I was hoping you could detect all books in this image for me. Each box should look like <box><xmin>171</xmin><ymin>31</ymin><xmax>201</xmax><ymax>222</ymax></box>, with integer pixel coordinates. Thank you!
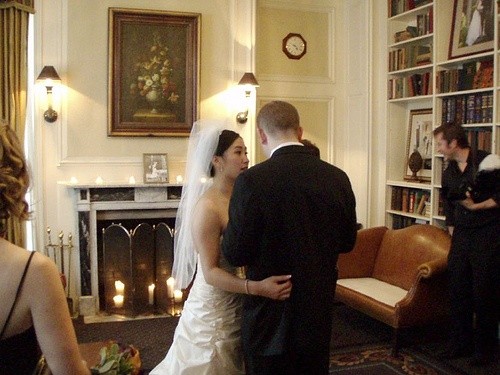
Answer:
<box><xmin>387</xmin><ymin>0</ymin><xmax>494</xmax><ymax>236</ymax></box>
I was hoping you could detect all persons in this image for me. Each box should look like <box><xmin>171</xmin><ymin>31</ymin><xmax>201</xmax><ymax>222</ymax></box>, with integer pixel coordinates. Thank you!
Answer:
<box><xmin>0</xmin><ymin>121</ymin><xmax>91</xmax><ymax>375</ymax></box>
<box><xmin>149</xmin><ymin>101</ymin><xmax>358</xmax><ymax>375</ymax></box>
<box><xmin>433</xmin><ymin>123</ymin><xmax>500</xmax><ymax>363</ymax></box>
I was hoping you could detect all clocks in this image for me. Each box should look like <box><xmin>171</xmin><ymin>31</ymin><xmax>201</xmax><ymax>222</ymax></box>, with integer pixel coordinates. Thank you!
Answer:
<box><xmin>281</xmin><ymin>32</ymin><xmax>307</xmax><ymax>60</ymax></box>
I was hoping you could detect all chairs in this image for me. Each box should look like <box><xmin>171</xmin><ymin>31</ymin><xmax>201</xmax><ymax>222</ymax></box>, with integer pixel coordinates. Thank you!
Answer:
<box><xmin>333</xmin><ymin>223</ymin><xmax>452</xmax><ymax>358</ymax></box>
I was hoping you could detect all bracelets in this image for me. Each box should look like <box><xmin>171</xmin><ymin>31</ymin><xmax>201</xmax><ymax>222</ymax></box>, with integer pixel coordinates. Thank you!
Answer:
<box><xmin>244</xmin><ymin>278</ymin><xmax>251</xmax><ymax>295</ymax></box>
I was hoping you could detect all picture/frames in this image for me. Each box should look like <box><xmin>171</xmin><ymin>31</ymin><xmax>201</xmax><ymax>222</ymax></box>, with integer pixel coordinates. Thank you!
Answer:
<box><xmin>403</xmin><ymin>108</ymin><xmax>433</xmax><ymax>183</ymax></box>
<box><xmin>448</xmin><ymin>0</ymin><xmax>495</xmax><ymax>61</ymax></box>
<box><xmin>104</xmin><ymin>6</ymin><xmax>203</xmax><ymax>139</ymax></box>
<box><xmin>143</xmin><ymin>152</ymin><xmax>169</xmax><ymax>184</ymax></box>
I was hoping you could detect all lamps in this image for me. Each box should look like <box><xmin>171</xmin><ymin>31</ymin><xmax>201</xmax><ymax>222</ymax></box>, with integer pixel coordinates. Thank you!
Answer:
<box><xmin>234</xmin><ymin>72</ymin><xmax>260</xmax><ymax>125</ymax></box>
<box><xmin>36</xmin><ymin>65</ymin><xmax>62</xmax><ymax>123</ymax></box>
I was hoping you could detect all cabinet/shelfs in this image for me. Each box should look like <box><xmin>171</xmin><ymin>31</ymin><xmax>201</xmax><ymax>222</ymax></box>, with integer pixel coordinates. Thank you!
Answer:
<box><xmin>385</xmin><ymin>0</ymin><xmax>500</xmax><ymax>230</ymax></box>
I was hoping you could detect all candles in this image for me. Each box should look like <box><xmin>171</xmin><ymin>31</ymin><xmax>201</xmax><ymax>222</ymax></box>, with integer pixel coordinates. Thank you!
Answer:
<box><xmin>165</xmin><ymin>278</ymin><xmax>175</xmax><ymax>299</ymax></box>
<box><xmin>113</xmin><ymin>295</ymin><xmax>124</xmax><ymax>308</ymax></box>
<box><xmin>172</xmin><ymin>290</ymin><xmax>183</xmax><ymax>303</ymax></box>
<box><xmin>114</xmin><ymin>280</ymin><xmax>125</xmax><ymax>295</ymax></box>
<box><xmin>147</xmin><ymin>284</ymin><xmax>155</xmax><ymax>305</ymax></box>
